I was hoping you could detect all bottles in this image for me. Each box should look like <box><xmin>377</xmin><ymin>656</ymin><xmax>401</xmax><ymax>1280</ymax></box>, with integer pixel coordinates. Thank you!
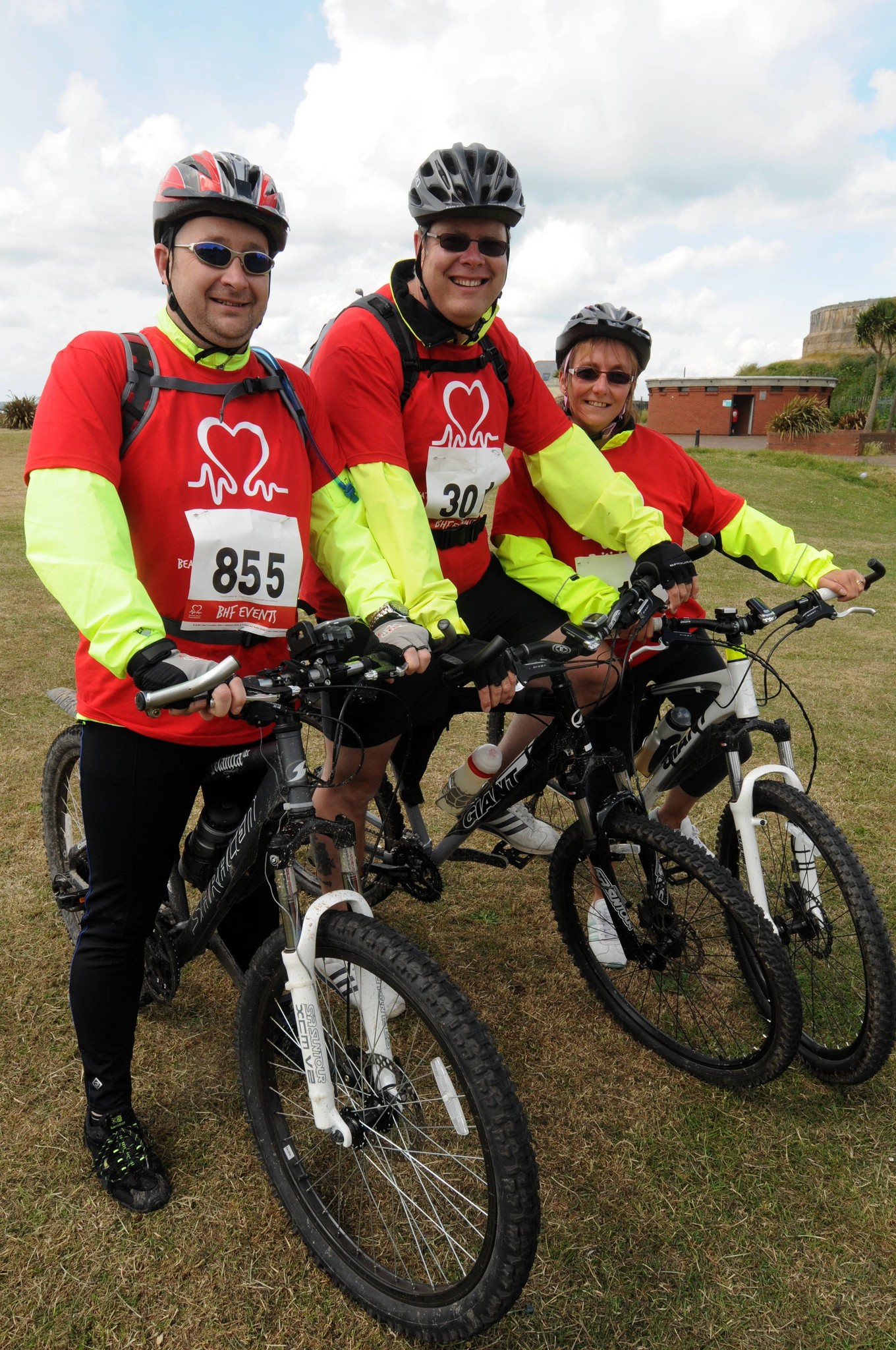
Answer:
<box><xmin>178</xmin><ymin>793</ymin><xmax>246</xmax><ymax>886</ymax></box>
<box><xmin>437</xmin><ymin>743</ymin><xmax>503</xmax><ymax>814</ymax></box>
<box><xmin>633</xmin><ymin>705</ymin><xmax>693</xmax><ymax>777</ymax></box>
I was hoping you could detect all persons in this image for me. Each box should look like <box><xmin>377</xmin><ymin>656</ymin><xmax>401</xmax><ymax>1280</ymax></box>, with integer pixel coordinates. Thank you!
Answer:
<box><xmin>491</xmin><ymin>304</ymin><xmax>865</xmax><ymax>968</ymax></box>
<box><xmin>732</xmin><ymin>405</ymin><xmax>738</xmax><ymax>436</ymax></box>
<box><xmin>299</xmin><ymin>143</ymin><xmax>699</xmax><ymax>1020</ymax></box>
<box><xmin>23</xmin><ymin>156</ymin><xmax>433</xmax><ymax>1212</ymax></box>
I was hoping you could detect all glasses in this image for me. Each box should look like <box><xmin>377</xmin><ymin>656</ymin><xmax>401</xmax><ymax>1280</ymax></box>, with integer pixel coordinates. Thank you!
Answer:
<box><xmin>170</xmin><ymin>241</ymin><xmax>274</xmax><ymax>275</ymax></box>
<box><xmin>568</xmin><ymin>366</ymin><xmax>635</xmax><ymax>385</ymax></box>
<box><xmin>426</xmin><ymin>232</ymin><xmax>510</xmax><ymax>257</ymax></box>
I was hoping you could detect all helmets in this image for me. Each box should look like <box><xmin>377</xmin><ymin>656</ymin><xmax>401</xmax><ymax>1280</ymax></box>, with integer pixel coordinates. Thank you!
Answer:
<box><xmin>408</xmin><ymin>141</ymin><xmax>526</xmax><ymax>227</ymax></box>
<box><xmin>555</xmin><ymin>303</ymin><xmax>652</xmax><ymax>377</ymax></box>
<box><xmin>154</xmin><ymin>151</ymin><xmax>289</xmax><ymax>258</ymax></box>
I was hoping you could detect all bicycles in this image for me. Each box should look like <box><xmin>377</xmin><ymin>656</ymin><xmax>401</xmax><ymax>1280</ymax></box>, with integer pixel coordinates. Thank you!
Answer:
<box><xmin>292</xmin><ymin>530</ymin><xmax>802</xmax><ymax>1085</ymax></box>
<box><xmin>487</xmin><ymin>558</ymin><xmax>896</xmax><ymax>1085</ymax></box>
<box><xmin>42</xmin><ymin>558</ymin><xmax>541</xmax><ymax>1344</ymax></box>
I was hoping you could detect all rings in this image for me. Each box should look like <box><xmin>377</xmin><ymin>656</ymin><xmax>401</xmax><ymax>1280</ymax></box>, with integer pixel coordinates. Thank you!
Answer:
<box><xmin>855</xmin><ymin>578</ymin><xmax>863</xmax><ymax>585</ymax></box>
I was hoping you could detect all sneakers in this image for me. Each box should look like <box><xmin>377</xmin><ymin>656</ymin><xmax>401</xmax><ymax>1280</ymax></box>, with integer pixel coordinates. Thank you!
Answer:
<box><xmin>180</xmin><ymin>829</ymin><xmax>221</xmax><ymax>888</ymax></box>
<box><xmin>648</xmin><ymin>807</ymin><xmax>716</xmax><ymax>858</ymax></box>
<box><xmin>83</xmin><ymin>1107</ymin><xmax>170</xmax><ymax>1215</ymax></box>
<box><xmin>476</xmin><ymin>800</ymin><xmax>560</xmax><ymax>855</ymax></box>
<box><xmin>314</xmin><ymin>956</ymin><xmax>404</xmax><ymax>1019</ymax></box>
<box><xmin>587</xmin><ymin>899</ymin><xmax>628</xmax><ymax>969</ymax></box>
